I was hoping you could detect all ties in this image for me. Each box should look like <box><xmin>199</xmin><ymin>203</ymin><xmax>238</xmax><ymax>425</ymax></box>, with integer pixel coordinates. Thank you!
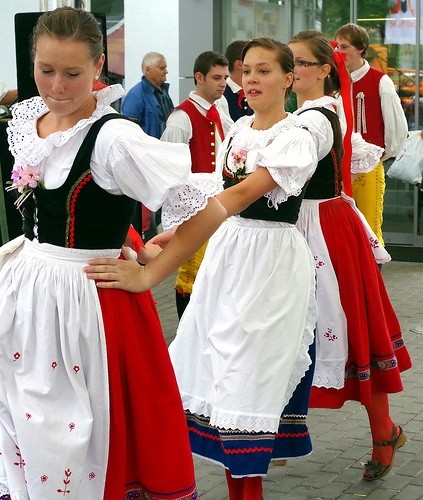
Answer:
<box><xmin>207</xmin><ymin>105</ymin><xmax>225</xmax><ymax>141</ymax></box>
<box><xmin>238</xmin><ymin>89</ymin><xmax>245</xmax><ymax>109</ymax></box>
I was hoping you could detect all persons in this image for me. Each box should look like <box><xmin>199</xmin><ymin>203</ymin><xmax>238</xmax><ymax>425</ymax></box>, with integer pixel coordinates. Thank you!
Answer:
<box><xmin>0</xmin><ymin>77</ymin><xmax>20</xmax><ymax>104</ymax></box>
<box><xmin>160</xmin><ymin>51</ymin><xmax>233</xmax><ymax>322</ymax></box>
<box><xmin>121</xmin><ymin>51</ymin><xmax>175</xmax><ymax>239</ymax></box>
<box><xmin>389</xmin><ymin>0</ymin><xmax>415</xmax><ymax>29</ymax></box>
<box><xmin>140</xmin><ymin>38</ymin><xmax>320</xmax><ymax>500</ymax></box>
<box><xmin>334</xmin><ymin>23</ymin><xmax>408</xmax><ymax>272</ymax></box>
<box><xmin>282</xmin><ymin>29</ymin><xmax>413</xmax><ymax>482</ymax></box>
<box><xmin>0</xmin><ymin>7</ymin><xmax>228</xmax><ymax>500</ymax></box>
<box><xmin>223</xmin><ymin>40</ymin><xmax>255</xmax><ymax>122</ymax></box>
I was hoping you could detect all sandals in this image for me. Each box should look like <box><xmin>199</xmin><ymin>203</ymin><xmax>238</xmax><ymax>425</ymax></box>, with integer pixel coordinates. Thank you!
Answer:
<box><xmin>363</xmin><ymin>425</ymin><xmax>406</xmax><ymax>481</ymax></box>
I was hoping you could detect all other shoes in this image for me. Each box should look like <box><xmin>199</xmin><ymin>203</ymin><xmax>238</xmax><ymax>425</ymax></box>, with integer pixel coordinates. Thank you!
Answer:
<box><xmin>272</xmin><ymin>460</ymin><xmax>287</xmax><ymax>466</ymax></box>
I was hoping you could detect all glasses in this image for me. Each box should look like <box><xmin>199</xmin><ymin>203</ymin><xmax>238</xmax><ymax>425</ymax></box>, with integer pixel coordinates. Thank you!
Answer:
<box><xmin>293</xmin><ymin>60</ymin><xmax>318</xmax><ymax>67</ymax></box>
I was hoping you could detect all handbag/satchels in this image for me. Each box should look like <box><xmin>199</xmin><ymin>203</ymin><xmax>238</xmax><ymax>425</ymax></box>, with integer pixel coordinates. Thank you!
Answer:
<box><xmin>389</xmin><ymin>130</ymin><xmax>423</xmax><ymax>183</ymax></box>
